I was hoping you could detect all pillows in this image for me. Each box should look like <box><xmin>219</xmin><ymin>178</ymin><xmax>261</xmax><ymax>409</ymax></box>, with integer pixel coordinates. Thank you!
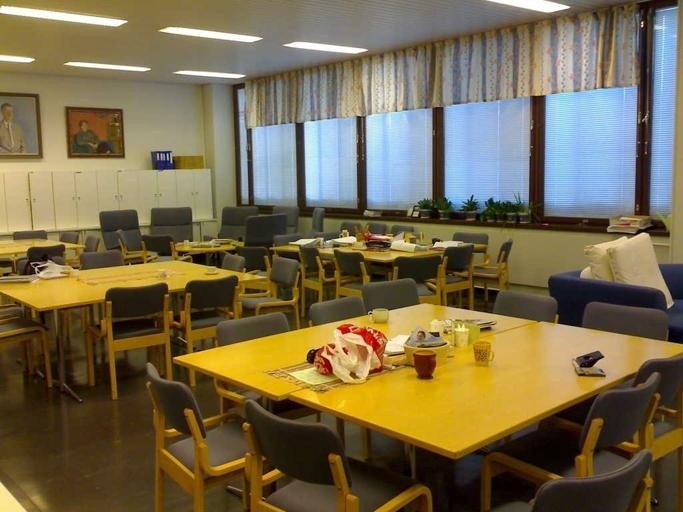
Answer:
<box><xmin>606</xmin><ymin>232</ymin><xmax>674</xmax><ymax>311</ymax></box>
<box><xmin>583</xmin><ymin>235</ymin><xmax>629</xmax><ymax>283</ymax></box>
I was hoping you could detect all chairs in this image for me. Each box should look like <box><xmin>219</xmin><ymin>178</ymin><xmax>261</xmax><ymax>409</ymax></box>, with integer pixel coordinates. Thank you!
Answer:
<box><xmin>0</xmin><ymin>206</ymin><xmax>513</xmax><ymax>400</ymax></box>
<box><xmin>142</xmin><ymin>278</ymin><xmax>682</xmax><ymax>511</ymax></box>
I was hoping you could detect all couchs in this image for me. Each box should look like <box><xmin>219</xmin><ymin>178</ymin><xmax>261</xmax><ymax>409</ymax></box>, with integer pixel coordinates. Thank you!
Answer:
<box><xmin>548</xmin><ymin>264</ymin><xmax>683</xmax><ymax>344</ymax></box>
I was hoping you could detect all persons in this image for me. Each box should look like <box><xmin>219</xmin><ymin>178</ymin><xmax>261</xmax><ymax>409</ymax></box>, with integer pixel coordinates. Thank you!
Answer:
<box><xmin>0</xmin><ymin>103</ymin><xmax>26</xmax><ymax>154</ymax></box>
<box><xmin>73</xmin><ymin>119</ymin><xmax>111</xmax><ymax>153</ymax></box>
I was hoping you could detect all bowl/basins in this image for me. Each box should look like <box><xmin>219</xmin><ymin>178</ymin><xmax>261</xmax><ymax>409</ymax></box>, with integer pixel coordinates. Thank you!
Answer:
<box><xmin>404</xmin><ymin>340</ymin><xmax>449</xmax><ymax>367</ymax></box>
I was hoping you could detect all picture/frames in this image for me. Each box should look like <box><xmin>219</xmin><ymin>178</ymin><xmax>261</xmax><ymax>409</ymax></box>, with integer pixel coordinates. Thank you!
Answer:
<box><xmin>64</xmin><ymin>106</ymin><xmax>125</xmax><ymax>158</ymax></box>
<box><xmin>0</xmin><ymin>91</ymin><xmax>43</xmax><ymax>159</ymax></box>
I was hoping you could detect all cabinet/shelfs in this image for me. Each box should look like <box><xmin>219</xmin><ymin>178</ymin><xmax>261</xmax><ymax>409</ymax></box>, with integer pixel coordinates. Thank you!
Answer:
<box><xmin>137</xmin><ymin>170</ymin><xmax>174</xmax><ymax>225</ymax></box>
<box><xmin>50</xmin><ymin>170</ymin><xmax>95</xmax><ymax>228</ymax></box>
<box><xmin>0</xmin><ymin>168</ymin><xmax>54</xmax><ymax>232</ymax></box>
<box><xmin>171</xmin><ymin>168</ymin><xmax>212</xmax><ymax>220</ymax></box>
<box><xmin>91</xmin><ymin>169</ymin><xmax>141</xmax><ymax>228</ymax></box>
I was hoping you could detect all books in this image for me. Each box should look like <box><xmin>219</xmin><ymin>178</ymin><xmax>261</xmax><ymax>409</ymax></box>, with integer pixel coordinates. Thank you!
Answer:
<box><xmin>151</xmin><ymin>150</ymin><xmax>174</xmax><ymax>169</ymax></box>
<box><xmin>364</xmin><ymin>209</ymin><xmax>383</xmax><ymax>219</ymax></box>
<box><xmin>606</xmin><ymin>216</ymin><xmax>650</xmax><ymax>235</ymax></box>
<box><xmin>0</xmin><ymin>274</ymin><xmax>38</xmax><ymax>284</ymax></box>
<box><xmin>288</xmin><ymin>230</ymin><xmax>465</xmax><ymax>256</ymax></box>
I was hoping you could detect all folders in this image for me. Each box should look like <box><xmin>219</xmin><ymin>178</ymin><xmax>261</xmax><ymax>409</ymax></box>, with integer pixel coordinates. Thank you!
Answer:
<box><xmin>151</xmin><ymin>150</ymin><xmax>174</xmax><ymax>170</ymax></box>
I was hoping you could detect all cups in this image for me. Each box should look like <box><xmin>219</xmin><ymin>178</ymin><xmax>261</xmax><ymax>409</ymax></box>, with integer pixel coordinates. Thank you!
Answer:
<box><xmin>472</xmin><ymin>341</ymin><xmax>494</xmax><ymax>367</ymax></box>
<box><xmin>455</xmin><ymin>328</ymin><xmax>469</xmax><ymax>346</ymax></box>
<box><xmin>207</xmin><ymin>267</ymin><xmax>215</xmax><ymax>271</ymax></box>
<box><xmin>160</xmin><ymin>270</ymin><xmax>168</xmax><ymax>277</ymax></box>
<box><xmin>183</xmin><ymin>240</ymin><xmax>189</xmax><ymax>247</ymax></box>
<box><xmin>339</xmin><ymin>227</ymin><xmax>349</xmax><ymax>238</ymax></box>
<box><xmin>355</xmin><ymin>231</ymin><xmax>444</xmax><ymax>252</ymax></box>
<box><xmin>317</xmin><ymin>237</ymin><xmax>324</xmax><ymax>247</ymax></box>
<box><xmin>367</xmin><ymin>308</ymin><xmax>388</xmax><ymax>323</ymax></box>
<box><xmin>413</xmin><ymin>349</ymin><xmax>436</xmax><ymax>377</ymax></box>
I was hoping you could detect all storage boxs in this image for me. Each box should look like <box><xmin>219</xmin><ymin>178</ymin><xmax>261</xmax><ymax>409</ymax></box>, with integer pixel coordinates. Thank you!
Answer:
<box><xmin>174</xmin><ymin>156</ymin><xmax>204</xmax><ymax>168</ymax></box>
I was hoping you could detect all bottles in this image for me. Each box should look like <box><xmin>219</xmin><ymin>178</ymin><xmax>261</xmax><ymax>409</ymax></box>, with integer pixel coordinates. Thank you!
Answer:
<box><xmin>442</xmin><ymin>326</ymin><xmax>452</xmax><ymax>345</ymax></box>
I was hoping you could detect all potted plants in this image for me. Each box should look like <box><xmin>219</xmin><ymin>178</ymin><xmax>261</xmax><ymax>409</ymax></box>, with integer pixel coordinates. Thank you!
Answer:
<box><xmin>413</xmin><ymin>193</ymin><xmax>532</xmax><ymax>227</ymax></box>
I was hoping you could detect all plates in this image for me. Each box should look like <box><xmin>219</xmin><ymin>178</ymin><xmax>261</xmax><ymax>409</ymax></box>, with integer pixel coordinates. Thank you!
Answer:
<box><xmin>214</xmin><ymin>239</ymin><xmax>232</xmax><ymax>243</ymax></box>
<box><xmin>203</xmin><ymin>271</ymin><xmax>219</xmax><ymax>274</ymax></box>
